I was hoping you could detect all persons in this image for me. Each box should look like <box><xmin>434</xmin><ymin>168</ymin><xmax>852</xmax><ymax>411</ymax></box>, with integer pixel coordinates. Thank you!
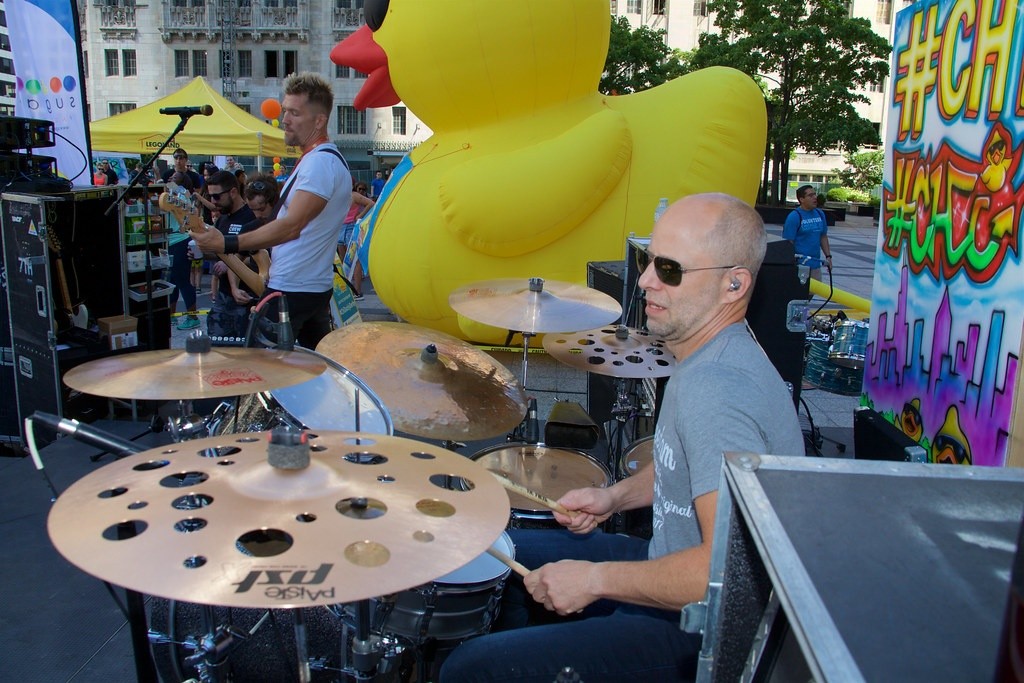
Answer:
<box><xmin>782</xmin><ymin>184</ymin><xmax>832</xmax><ymax>282</ymax></box>
<box><xmin>440</xmin><ymin>192</ymin><xmax>804</xmax><ymax>683</ymax></box>
<box><xmin>188</xmin><ymin>71</ymin><xmax>352</xmax><ymax>350</ymax></box>
<box><xmin>93</xmin><ymin>158</ymin><xmax>119</xmax><ymax>186</ymax></box>
<box><xmin>338</xmin><ymin>169</ymin><xmax>398</xmax><ymax>301</ymax></box>
<box><xmin>127</xmin><ymin>162</ymin><xmax>145</xmax><ymax>186</ymax></box>
<box><xmin>161</xmin><ymin>148</ymin><xmax>291</xmax><ymax>340</ymax></box>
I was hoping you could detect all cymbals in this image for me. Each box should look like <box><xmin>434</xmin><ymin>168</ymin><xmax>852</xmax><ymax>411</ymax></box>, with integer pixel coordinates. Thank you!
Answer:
<box><xmin>447</xmin><ymin>277</ymin><xmax>624</xmax><ymax>336</ymax></box>
<box><xmin>317</xmin><ymin>321</ymin><xmax>528</xmax><ymax>441</ymax></box>
<box><xmin>47</xmin><ymin>428</ymin><xmax>510</xmax><ymax>609</ymax></box>
<box><xmin>541</xmin><ymin>324</ymin><xmax>679</xmax><ymax>379</ymax></box>
<box><xmin>62</xmin><ymin>348</ymin><xmax>328</xmax><ymax>401</ymax></box>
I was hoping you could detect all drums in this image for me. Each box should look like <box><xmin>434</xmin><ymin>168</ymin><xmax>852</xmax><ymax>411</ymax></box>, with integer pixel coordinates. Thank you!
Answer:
<box><xmin>147</xmin><ymin>587</ymin><xmax>413</xmax><ymax>683</ymax></box>
<box><xmin>620</xmin><ymin>433</ymin><xmax>654</xmax><ymax>476</ymax></box>
<box><xmin>322</xmin><ymin>528</ymin><xmax>517</xmax><ymax>641</ymax></box>
<box><xmin>826</xmin><ymin>320</ymin><xmax>870</xmax><ymax>366</ymax></box>
<box><xmin>206</xmin><ymin>344</ymin><xmax>395</xmax><ymax>459</ymax></box>
<box><xmin>801</xmin><ymin>335</ymin><xmax>865</xmax><ymax>398</ymax></box>
<box><xmin>459</xmin><ymin>443</ymin><xmax>613</xmax><ymax>530</ymax></box>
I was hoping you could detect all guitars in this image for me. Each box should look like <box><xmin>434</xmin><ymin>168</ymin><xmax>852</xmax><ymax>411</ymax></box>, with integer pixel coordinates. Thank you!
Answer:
<box><xmin>156</xmin><ymin>189</ymin><xmax>266</xmax><ymax>297</ymax></box>
<box><xmin>45</xmin><ymin>227</ymin><xmax>90</xmax><ymax>333</ymax></box>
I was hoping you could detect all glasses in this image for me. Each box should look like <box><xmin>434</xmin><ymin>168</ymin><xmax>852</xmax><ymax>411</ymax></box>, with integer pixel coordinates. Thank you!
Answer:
<box><xmin>636</xmin><ymin>247</ymin><xmax>736</xmax><ymax>286</ymax></box>
<box><xmin>206</xmin><ymin>185</ymin><xmax>238</xmax><ymax>201</ymax></box>
<box><xmin>98</xmin><ymin>168</ymin><xmax>104</xmax><ymax>170</ymax></box>
<box><xmin>359</xmin><ymin>188</ymin><xmax>365</xmax><ymax>191</ymax></box>
<box><xmin>245</xmin><ymin>182</ymin><xmax>266</xmax><ymax>191</ymax></box>
<box><xmin>102</xmin><ymin>162</ymin><xmax>108</xmax><ymax>164</ymax></box>
<box><xmin>173</xmin><ymin>154</ymin><xmax>187</xmax><ymax>159</ymax></box>
<box><xmin>806</xmin><ymin>192</ymin><xmax>816</xmax><ymax>198</ymax></box>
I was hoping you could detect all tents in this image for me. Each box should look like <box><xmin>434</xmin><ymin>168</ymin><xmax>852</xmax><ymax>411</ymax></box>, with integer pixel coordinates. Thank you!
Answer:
<box><xmin>88</xmin><ymin>76</ymin><xmax>304</xmax><ymax>174</ymax></box>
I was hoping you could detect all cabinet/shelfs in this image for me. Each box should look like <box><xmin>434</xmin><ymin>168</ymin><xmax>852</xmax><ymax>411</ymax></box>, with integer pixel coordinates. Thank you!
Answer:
<box><xmin>118</xmin><ymin>183</ymin><xmax>176</xmax><ymax>316</ymax></box>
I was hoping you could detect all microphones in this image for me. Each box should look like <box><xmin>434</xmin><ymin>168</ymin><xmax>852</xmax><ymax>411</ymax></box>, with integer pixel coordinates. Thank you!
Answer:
<box><xmin>32</xmin><ymin>411</ymin><xmax>166</xmax><ymax>467</ymax></box>
<box><xmin>279</xmin><ymin>295</ymin><xmax>295</xmax><ymax>352</ymax></box>
<box><xmin>527</xmin><ymin>399</ymin><xmax>539</xmax><ymax>444</ymax></box>
<box><xmin>159</xmin><ymin>105</ymin><xmax>213</xmax><ymax>116</ymax></box>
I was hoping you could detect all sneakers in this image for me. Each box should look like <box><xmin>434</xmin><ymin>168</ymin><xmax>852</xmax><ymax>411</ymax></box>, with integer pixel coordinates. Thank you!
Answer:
<box><xmin>171</xmin><ymin>316</ymin><xmax>178</xmax><ymax>326</ymax></box>
<box><xmin>177</xmin><ymin>317</ymin><xmax>201</xmax><ymax>330</ymax></box>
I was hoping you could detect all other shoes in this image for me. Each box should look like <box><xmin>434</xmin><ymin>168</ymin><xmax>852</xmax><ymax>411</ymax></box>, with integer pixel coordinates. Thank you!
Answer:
<box><xmin>353</xmin><ymin>292</ymin><xmax>365</xmax><ymax>301</ymax></box>
<box><xmin>196</xmin><ymin>288</ymin><xmax>202</xmax><ymax>294</ymax></box>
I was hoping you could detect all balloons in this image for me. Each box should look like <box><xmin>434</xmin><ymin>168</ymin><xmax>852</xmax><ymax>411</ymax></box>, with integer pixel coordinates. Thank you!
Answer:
<box><xmin>261</xmin><ymin>99</ymin><xmax>281</xmax><ymax>177</ymax></box>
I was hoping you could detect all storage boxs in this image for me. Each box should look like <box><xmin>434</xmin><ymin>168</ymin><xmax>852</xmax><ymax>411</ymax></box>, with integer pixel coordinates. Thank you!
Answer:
<box><xmin>99</xmin><ymin>315</ymin><xmax>138</xmax><ymax>351</ymax></box>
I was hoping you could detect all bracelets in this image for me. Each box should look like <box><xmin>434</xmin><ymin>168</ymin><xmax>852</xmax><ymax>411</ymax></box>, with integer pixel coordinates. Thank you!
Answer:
<box><xmin>223</xmin><ymin>234</ymin><xmax>239</xmax><ymax>256</ymax></box>
<box><xmin>826</xmin><ymin>254</ymin><xmax>832</xmax><ymax>259</ymax></box>
